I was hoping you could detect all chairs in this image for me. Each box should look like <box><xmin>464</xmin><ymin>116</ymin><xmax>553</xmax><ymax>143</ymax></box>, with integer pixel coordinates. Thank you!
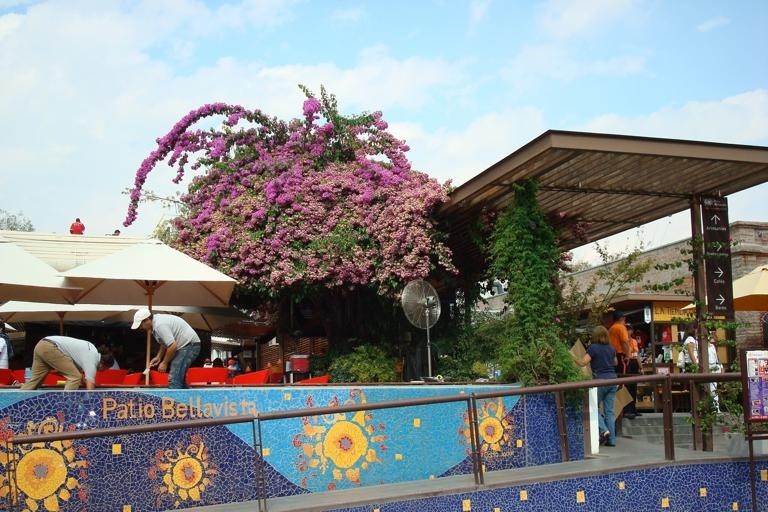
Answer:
<box><xmin>0</xmin><ymin>367</ymin><xmax>329</xmax><ymax>385</ymax></box>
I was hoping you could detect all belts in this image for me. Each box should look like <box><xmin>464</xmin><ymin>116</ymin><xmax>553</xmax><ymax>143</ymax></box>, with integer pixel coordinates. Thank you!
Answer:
<box><xmin>43</xmin><ymin>338</ymin><xmax>56</xmax><ymax>346</ymax></box>
<box><xmin>186</xmin><ymin>342</ymin><xmax>201</xmax><ymax>346</ymax></box>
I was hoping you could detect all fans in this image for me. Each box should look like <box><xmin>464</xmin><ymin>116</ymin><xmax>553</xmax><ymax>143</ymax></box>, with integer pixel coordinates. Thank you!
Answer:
<box><xmin>401</xmin><ymin>279</ymin><xmax>442</xmax><ymax>380</ymax></box>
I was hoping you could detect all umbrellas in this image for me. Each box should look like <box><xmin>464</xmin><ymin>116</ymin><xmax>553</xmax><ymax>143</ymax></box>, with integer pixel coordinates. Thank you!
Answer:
<box><xmin>59</xmin><ymin>236</ymin><xmax>238</xmax><ymax>384</ymax></box>
<box><xmin>0</xmin><ymin>299</ymin><xmax>130</xmax><ymax>337</ymax></box>
<box><xmin>0</xmin><ymin>236</ymin><xmax>83</xmax><ymax>330</ymax></box>
<box><xmin>110</xmin><ymin>303</ymin><xmax>250</xmax><ymax>331</ymax></box>
<box><xmin>679</xmin><ymin>263</ymin><xmax>768</xmax><ymax>312</ymax></box>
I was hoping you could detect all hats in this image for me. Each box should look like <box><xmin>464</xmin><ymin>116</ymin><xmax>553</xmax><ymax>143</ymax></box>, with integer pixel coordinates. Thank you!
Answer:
<box><xmin>131</xmin><ymin>306</ymin><xmax>151</xmax><ymax>331</ymax></box>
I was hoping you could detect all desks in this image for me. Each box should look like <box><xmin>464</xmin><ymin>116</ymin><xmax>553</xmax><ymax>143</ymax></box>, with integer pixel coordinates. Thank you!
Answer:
<box><xmin>659</xmin><ymin>390</ymin><xmax>689</xmax><ymax>413</ymax></box>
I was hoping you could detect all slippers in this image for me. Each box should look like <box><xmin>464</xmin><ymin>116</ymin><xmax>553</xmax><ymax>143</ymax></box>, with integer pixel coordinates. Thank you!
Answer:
<box><xmin>599</xmin><ymin>432</ymin><xmax>616</xmax><ymax>446</ymax></box>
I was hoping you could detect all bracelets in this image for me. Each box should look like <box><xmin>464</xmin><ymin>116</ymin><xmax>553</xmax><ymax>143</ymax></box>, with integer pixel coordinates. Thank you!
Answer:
<box><xmin>639</xmin><ymin>367</ymin><xmax>642</xmax><ymax>370</ymax></box>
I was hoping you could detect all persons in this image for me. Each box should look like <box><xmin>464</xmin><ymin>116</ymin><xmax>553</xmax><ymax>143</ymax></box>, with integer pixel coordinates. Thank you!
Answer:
<box><xmin>677</xmin><ymin>324</ymin><xmax>700</xmax><ymax>380</ymax></box>
<box><xmin>0</xmin><ymin>338</ymin><xmax>9</xmax><ymax>370</ymax></box>
<box><xmin>568</xmin><ymin>325</ymin><xmax>618</xmax><ymax>446</ymax></box>
<box><xmin>624</xmin><ymin>322</ymin><xmax>644</xmax><ymax>416</ymax></box>
<box><xmin>203</xmin><ymin>355</ymin><xmax>252</xmax><ymax>385</ymax></box>
<box><xmin>0</xmin><ymin>321</ymin><xmax>15</xmax><ymax>359</ymax></box>
<box><xmin>114</xmin><ymin>229</ymin><xmax>120</xmax><ymax>236</ymax></box>
<box><xmin>19</xmin><ymin>335</ymin><xmax>114</xmax><ymax>390</ymax></box>
<box><xmin>70</xmin><ymin>218</ymin><xmax>85</xmax><ymax>235</ymax></box>
<box><xmin>606</xmin><ymin>310</ymin><xmax>635</xmax><ymax>419</ymax></box>
<box><xmin>706</xmin><ymin>335</ymin><xmax>723</xmax><ymax>413</ymax></box>
<box><xmin>130</xmin><ymin>308</ymin><xmax>202</xmax><ymax>389</ymax></box>
